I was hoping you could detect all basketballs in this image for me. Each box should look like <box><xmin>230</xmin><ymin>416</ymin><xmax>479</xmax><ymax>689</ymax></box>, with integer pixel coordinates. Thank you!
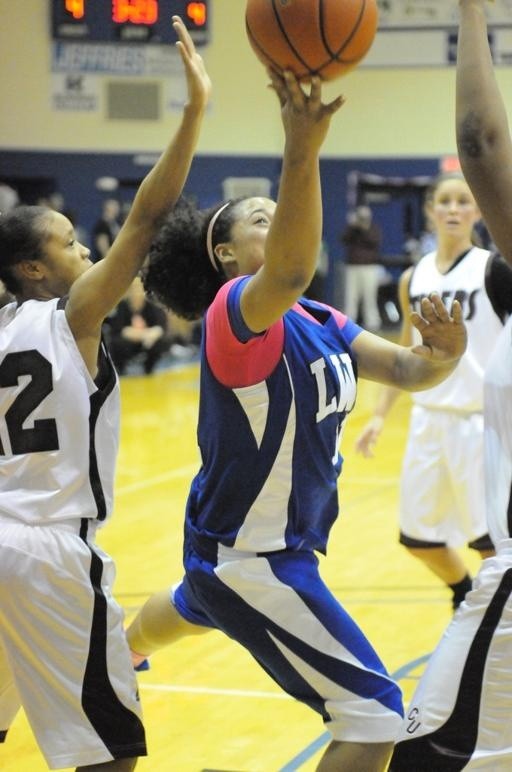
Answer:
<box><xmin>245</xmin><ymin>0</ymin><xmax>378</xmax><ymax>86</ymax></box>
